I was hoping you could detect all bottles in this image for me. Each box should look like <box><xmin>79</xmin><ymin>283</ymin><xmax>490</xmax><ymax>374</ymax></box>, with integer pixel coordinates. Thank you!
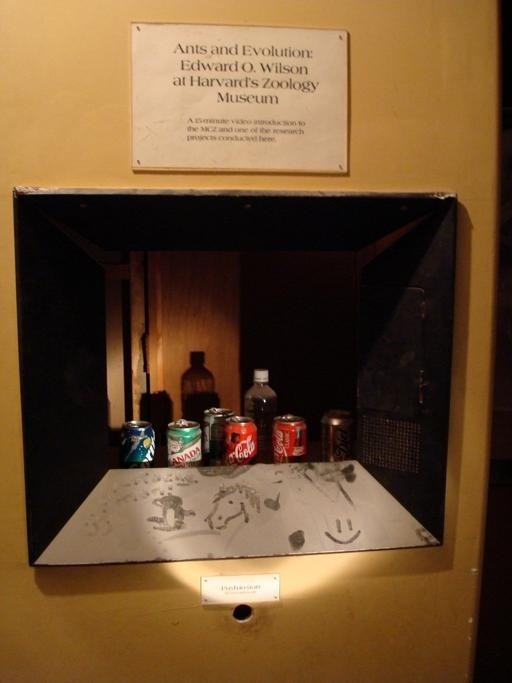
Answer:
<box><xmin>245</xmin><ymin>369</ymin><xmax>279</xmax><ymax>447</ymax></box>
<box><xmin>180</xmin><ymin>350</ymin><xmax>215</xmax><ymax>414</ymax></box>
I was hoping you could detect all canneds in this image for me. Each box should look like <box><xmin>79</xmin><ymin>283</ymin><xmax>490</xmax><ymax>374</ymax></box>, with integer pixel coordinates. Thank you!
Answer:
<box><xmin>166</xmin><ymin>418</ymin><xmax>204</xmax><ymax>467</ymax></box>
<box><xmin>271</xmin><ymin>415</ymin><xmax>311</xmax><ymax>462</ymax></box>
<box><xmin>203</xmin><ymin>408</ymin><xmax>234</xmax><ymax>454</ymax></box>
<box><xmin>120</xmin><ymin>420</ymin><xmax>158</xmax><ymax>468</ymax></box>
<box><xmin>317</xmin><ymin>408</ymin><xmax>355</xmax><ymax>461</ymax></box>
<box><xmin>223</xmin><ymin>415</ymin><xmax>260</xmax><ymax>465</ymax></box>
